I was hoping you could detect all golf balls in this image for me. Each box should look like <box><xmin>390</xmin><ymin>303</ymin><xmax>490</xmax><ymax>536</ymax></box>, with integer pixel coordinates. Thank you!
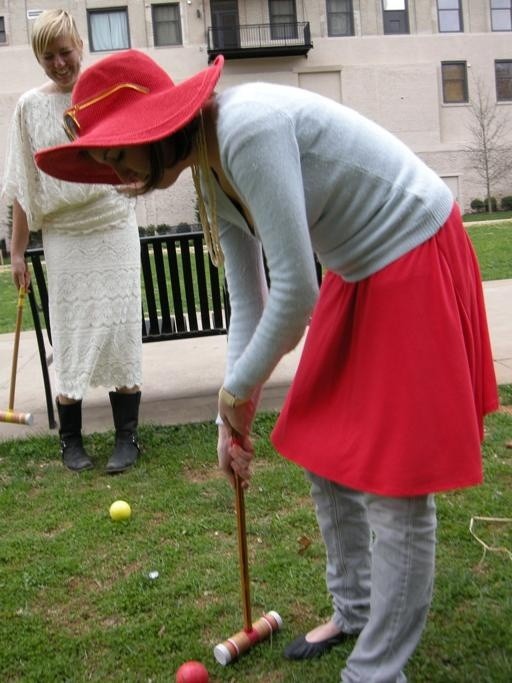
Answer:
<box><xmin>110</xmin><ymin>501</ymin><xmax>131</xmax><ymax>520</ymax></box>
<box><xmin>176</xmin><ymin>661</ymin><xmax>209</xmax><ymax>682</ymax></box>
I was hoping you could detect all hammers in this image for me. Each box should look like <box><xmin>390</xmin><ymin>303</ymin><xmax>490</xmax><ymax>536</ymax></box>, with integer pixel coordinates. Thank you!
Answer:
<box><xmin>213</xmin><ymin>468</ymin><xmax>284</xmax><ymax>666</ymax></box>
<box><xmin>0</xmin><ymin>285</ymin><xmax>36</xmax><ymax>425</ymax></box>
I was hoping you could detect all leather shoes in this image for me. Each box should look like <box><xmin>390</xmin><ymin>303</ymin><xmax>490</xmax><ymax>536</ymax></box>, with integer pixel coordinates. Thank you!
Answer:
<box><xmin>280</xmin><ymin>622</ymin><xmax>357</xmax><ymax>660</ymax></box>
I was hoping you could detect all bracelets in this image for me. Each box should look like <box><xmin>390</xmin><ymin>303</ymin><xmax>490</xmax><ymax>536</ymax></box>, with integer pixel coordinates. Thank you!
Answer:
<box><xmin>218</xmin><ymin>388</ymin><xmax>247</xmax><ymax>407</ymax></box>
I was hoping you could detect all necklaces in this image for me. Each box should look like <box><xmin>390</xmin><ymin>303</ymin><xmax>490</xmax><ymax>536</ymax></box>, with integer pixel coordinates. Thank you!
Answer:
<box><xmin>190</xmin><ymin>106</ymin><xmax>226</xmax><ymax>267</ymax></box>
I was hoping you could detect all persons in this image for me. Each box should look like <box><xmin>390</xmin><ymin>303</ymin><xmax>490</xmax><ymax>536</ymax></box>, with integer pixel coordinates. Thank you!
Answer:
<box><xmin>35</xmin><ymin>48</ymin><xmax>498</xmax><ymax>682</ymax></box>
<box><xmin>2</xmin><ymin>7</ymin><xmax>144</xmax><ymax>473</ymax></box>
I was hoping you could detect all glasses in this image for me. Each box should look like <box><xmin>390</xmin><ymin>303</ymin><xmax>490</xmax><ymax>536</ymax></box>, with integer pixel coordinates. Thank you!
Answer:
<box><xmin>61</xmin><ymin>81</ymin><xmax>151</xmax><ymax>143</ymax></box>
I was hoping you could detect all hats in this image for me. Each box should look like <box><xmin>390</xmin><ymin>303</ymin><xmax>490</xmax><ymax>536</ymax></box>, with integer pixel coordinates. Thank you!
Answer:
<box><xmin>34</xmin><ymin>49</ymin><xmax>225</xmax><ymax>185</ymax></box>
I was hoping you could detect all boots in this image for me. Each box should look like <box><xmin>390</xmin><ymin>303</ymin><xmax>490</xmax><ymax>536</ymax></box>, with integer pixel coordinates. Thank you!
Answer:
<box><xmin>56</xmin><ymin>394</ymin><xmax>94</xmax><ymax>472</ymax></box>
<box><xmin>104</xmin><ymin>391</ymin><xmax>142</xmax><ymax>474</ymax></box>
